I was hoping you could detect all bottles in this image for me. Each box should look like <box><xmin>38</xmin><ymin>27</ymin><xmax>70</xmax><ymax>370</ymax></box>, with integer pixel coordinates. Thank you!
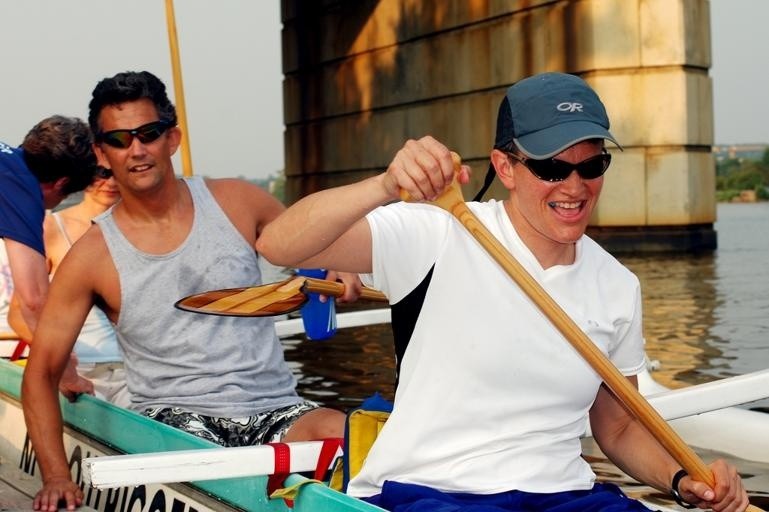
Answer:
<box><xmin>292</xmin><ymin>269</ymin><xmax>340</xmax><ymax>343</ymax></box>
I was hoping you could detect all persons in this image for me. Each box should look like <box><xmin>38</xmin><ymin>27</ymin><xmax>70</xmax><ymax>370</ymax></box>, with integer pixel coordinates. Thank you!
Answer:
<box><xmin>256</xmin><ymin>69</ymin><xmax>751</xmax><ymax>510</ymax></box>
<box><xmin>20</xmin><ymin>74</ymin><xmax>361</xmax><ymax>512</ymax></box>
<box><xmin>1</xmin><ymin>115</ymin><xmax>97</xmax><ymax>405</ymax></box>
<box><xmin>6</xmin><ymin>135</ymin><xmax>124</xmax><ymax>403</ymax></box>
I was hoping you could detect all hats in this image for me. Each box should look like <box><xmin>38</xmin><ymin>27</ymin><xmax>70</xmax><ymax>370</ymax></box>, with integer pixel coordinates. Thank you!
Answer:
<box><xmin>494</xmin><ymin>71</ymin><xmax>623</xmax><ymax>160</ymax></box>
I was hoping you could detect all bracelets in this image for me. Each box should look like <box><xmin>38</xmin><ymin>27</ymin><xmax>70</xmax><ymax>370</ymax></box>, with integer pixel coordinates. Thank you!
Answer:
<box><xmin>672</xmin><ymin>469</ymin><xmax>699</xmax><ymax>510</ymax></box>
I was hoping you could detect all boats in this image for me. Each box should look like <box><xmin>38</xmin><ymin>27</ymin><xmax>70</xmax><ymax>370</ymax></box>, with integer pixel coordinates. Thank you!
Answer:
<box><xmin>0</xmin><ymin>308</ymin><xmax>768</xmax><ymax>512</ymax></box>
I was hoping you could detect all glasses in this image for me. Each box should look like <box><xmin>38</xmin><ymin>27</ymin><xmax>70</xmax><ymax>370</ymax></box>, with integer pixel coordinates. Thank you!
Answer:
<box><xmin>98</xmin><ymin>119</ymin><xmax>169</xmax><ymax>148</ymax></box>
<box><xmin>96</xmin><ymin>165</ymin><xmax>113</xmax><ymax>178</ymax></box>
<box><xmin>503</xmin><ymin>148</ymin><xmax>611</xmax><ymax>182</ymax></box>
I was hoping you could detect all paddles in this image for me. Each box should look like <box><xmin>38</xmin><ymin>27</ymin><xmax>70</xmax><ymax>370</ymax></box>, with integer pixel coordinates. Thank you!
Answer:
<box><xmin>174</xmin><ymin>277</ymin><xmax>389</xmax><ymax>316</ymax></box>
